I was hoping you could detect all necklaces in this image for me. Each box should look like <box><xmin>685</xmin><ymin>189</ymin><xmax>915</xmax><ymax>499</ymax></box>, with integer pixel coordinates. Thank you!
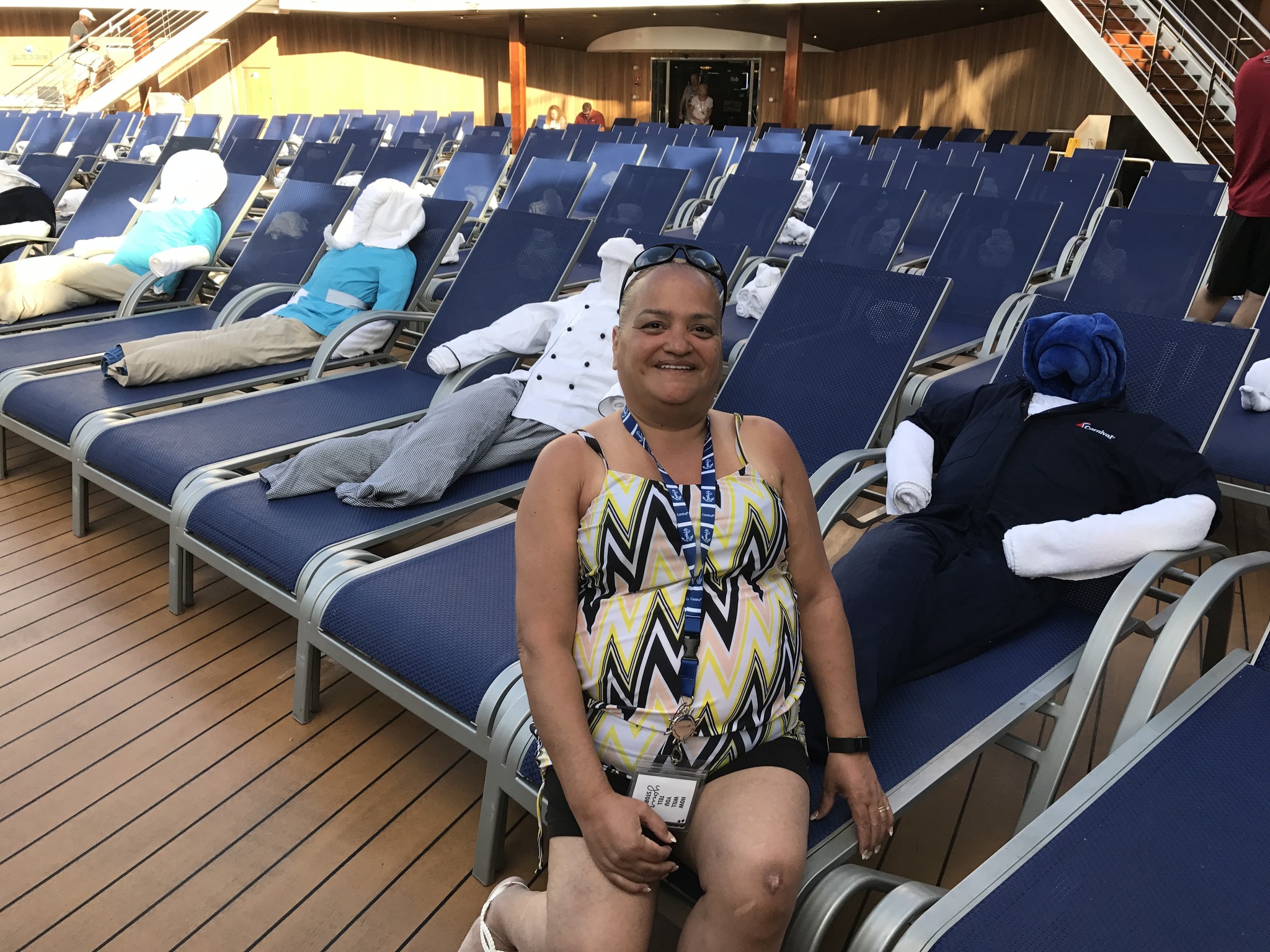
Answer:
<box><xmin>700</xmin><ymin>95</ymin><xmax>706</xmax><ymax>114</ymax></box>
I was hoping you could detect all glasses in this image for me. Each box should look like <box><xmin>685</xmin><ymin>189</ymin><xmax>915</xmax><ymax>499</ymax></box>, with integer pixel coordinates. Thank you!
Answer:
<box><xmin>619</xmin><ymin>243</ymin><xmax>727</xmax><ymax>322</ymax></box>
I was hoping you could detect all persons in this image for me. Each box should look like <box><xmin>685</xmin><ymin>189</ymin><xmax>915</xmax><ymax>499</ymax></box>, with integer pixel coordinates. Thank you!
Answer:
<box><xmin>455</xmin><ymin>244</ymin><xmax>895</xmax><ymax>952</ymax></box>
<box><xmin>678</xmin><ymin>73</ymin><xmax>713</xmax><ymax>126</ymax></box>
<box><xmin>543</xmin><ymin>105</ymin><xmax>567</xmax><ymax>129</ymax></box>
<box><xmin>1186</xmin><ymin>50</ymin><xmax>1270</xmax><ymax>330</ymax></box>
<box><xmin>574</xmin><ymin>102</ymin><xmax>605</xmax><ymax>132</ymax></box>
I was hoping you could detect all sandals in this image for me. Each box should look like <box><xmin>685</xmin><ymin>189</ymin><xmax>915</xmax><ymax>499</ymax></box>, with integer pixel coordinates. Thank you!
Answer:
<box><xmin>481</xmin><ymin>881</ymin><xmax>529</xmax><ymax>951</ymax></box>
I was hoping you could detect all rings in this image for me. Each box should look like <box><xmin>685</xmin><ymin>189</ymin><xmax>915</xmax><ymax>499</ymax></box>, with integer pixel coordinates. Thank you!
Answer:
<box><xmin>879</xmin><ymin>804</ymin><xmax>890</xmax><ymax>813</ymax></box>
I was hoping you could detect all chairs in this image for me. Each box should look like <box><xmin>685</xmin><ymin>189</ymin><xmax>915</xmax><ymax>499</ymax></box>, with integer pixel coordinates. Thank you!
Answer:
<box><xmin>0</xmin><ymin>109</ymin><xmax>1270</xmax><ymax>952</ymax></box>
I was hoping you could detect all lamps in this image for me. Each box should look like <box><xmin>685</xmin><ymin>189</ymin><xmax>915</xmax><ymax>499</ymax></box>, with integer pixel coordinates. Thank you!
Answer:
<box><xmin>684</xmin><ymin>54</ymin><xmax>689</xmax><ymax>59</ymax></box>
<box><xmin>720</xmin><ymin>54</ymin><xmax>726</xmax><ymax>58</ymax></box>
<box><xmin>769</xmin><ymin>97</ymin><xmax>775</xmax><ymax>102</ymax></box>
<box><xmin>770</xmin><ymin>66</ymin><xmax>776</xmax><ymax>71</ymax></box>
<box><xmin>633</xmin><ymin>94</ymin><xmax>639</xmax><ymax>100</ymax></box>
<box><xmin>633</xmin><ymin>65</ymin><xmax>639</xmax><ymax>71</ymax></box>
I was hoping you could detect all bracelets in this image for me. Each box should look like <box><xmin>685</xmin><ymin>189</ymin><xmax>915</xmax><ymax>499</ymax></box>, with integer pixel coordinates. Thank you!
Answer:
<box><xmin>825</xmin><ymin>736</ymin><xmax>874</xmax><ymax>753</ymax></box>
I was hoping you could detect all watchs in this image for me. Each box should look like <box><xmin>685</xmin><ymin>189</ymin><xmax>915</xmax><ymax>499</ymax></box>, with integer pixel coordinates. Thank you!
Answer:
<box><xmin>702</xmin><ymin>120</ymin><xmax>704</xmax><ymax>124</ymax></box>
